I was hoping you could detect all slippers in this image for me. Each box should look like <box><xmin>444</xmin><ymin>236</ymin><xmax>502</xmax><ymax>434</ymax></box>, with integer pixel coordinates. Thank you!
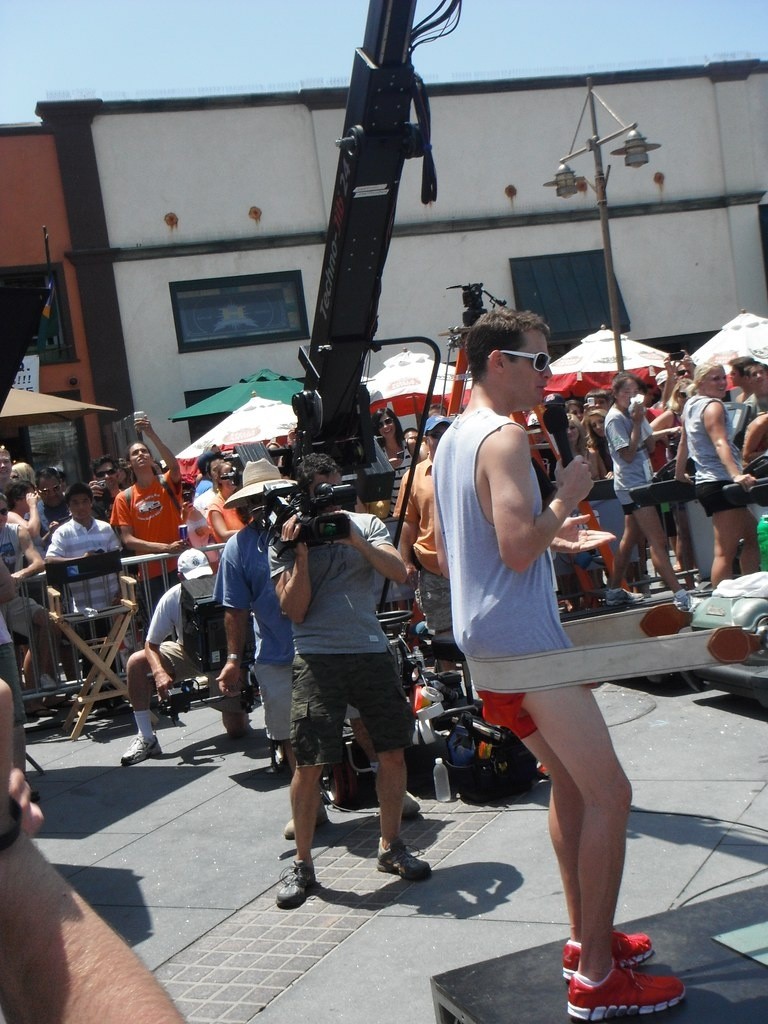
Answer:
<box><xmin>28</xmin><ymin>708</ymin><xmax>57</xmax><ymax>717</ymax></box>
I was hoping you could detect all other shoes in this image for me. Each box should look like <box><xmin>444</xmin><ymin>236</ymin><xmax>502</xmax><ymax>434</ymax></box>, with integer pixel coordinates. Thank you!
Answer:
<box><xmin>284</xmin><ymin>797</ymin><xmax>327</xmax><ymax>838</ymax></box>
<box><xmin>402</xmin><ymin>791</ymin><xmax>420</xmax><ymax>816</ymax></box>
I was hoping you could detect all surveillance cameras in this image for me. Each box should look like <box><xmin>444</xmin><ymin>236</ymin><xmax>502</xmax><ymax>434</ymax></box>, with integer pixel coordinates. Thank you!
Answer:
<box><xmin>69</xmin><ymin>377</ymin><xmax>78</xmax><ymax>386</ymax></box>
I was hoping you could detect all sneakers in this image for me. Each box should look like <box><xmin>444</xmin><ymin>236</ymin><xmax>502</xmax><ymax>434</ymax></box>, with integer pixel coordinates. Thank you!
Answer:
<box><xmin>276</xmin><ymin>859</ymin><xmax>316</xmax><ymax>909</ymax></box>
<box><xmin>567</xmin><ymin>956</ymin><xmax>685</xmax><ymax>1020</ymax></box>
<box><xmin>562</xmin><ymin>927</ymin><xmax>653</xmax><ymax>980</ymax></box>
<box><xmin>120</xmin><ymin>731</ymin><xmax>161</xmax><ymax>765</ymax></box>
<box><xmin>605</xmin><ymin>588</ymin><xmax>643</xmax><ymax>605</ymax></box>
<box><xmin>376</xmin><ymin>837</ymin><xmax>431</xmax><ymax>880</ymax></box>
<box><xmin>673</xmin><ymin>593</ymin><xmax>704</xmax><ymax>612</ymax></box>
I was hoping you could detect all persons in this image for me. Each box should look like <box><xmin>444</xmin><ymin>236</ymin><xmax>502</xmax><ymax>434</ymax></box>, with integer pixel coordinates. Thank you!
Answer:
<box><xmin>371</xmin><ymin>405</ymin><xmax>459</xmax><ymax>669</ymax></box>
<box><xmin>431</xmin><ymin>309</ymin><xmax>684</xmax><ymax>1022</ymax></box>
<box><xmin>1</xmin><ymin>414</ymin><xmax>420</xmax><ymax>837</ymax></box>
<box><xmin>268</xmin><ymin>455</ymin><xmax>429</xmax><ymax>907</ymax></box>
<box><xmin>0</xmin><ymin>678</ymin><xmax>187</xmax><ymax>1023</ymax></box>
<box><xmin>522</xmin><ymin>349</ymin><xmax>768</xmax><ymax>611</ymax></box>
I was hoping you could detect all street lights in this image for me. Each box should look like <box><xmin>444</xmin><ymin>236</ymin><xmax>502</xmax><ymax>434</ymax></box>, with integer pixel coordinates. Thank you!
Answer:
<box><xmin>542</xmin><ymin>76</ymin><xmax>661</xmax><ymax>372</ymax></box>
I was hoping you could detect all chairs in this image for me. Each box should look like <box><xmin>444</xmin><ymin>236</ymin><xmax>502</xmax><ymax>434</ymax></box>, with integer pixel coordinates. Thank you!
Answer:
<box><xmin>45</xmin><ymin>549</ymin><xmax>160</xmax><ymax>740</ymax></box>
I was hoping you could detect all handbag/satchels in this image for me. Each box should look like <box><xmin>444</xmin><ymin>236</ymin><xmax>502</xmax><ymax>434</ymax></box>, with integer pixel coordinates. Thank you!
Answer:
<box><xmin>444</xmin><ymin>711</ymin><xmax>536</xmax><ymax>803</ymax></box>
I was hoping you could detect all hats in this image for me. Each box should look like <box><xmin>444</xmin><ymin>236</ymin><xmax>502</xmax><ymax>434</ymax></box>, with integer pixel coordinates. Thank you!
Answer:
<box><xmin>424</xmin><ymin>415</ymin><xmax>451</xmax><ymax>436</ymax></box>
<box><xmin>177</xmin><ymin>548</ymin><xmax>213</xmax><ymax>580</ymax></box>
<box><xmin>197</xmin><ymin>451</ymin><xmax>217</xmax><ymax>473</ymax></box>
<box><xmin>224</xmin><ymin>459</ymin><xmax>297</xmax><ymax>509</ymax></box>
<box><xmin>543</xmin><ymin>393</ymin><xmax>565</xmax><ymax>405</ymax></box>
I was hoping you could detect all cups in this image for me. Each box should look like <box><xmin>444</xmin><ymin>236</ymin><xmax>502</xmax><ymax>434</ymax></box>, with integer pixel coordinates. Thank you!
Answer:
<box><xmin>179</xmin><ymin>524</ymin><xmax>188</xmax><ymax>545</ymax></box>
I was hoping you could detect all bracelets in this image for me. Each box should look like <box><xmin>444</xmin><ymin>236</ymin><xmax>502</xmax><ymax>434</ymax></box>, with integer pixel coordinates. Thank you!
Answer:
<box><xmin>226</xmin><ymin>653</ymin><xmax>240</xmax><ymax>660</ymax></box>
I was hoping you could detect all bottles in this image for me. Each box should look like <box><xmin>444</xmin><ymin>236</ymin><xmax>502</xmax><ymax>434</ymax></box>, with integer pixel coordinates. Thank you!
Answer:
<box><xmin>756</xmin><ymin>514</ymin><xmax>768</xmax><ymax>572</ymax></box>
<box><xmin>413</xmin><ymin>646</ymin><xmax>425</xmax><ymax>670</ymax></box>
<box><xmin>82</xmin><ymin>607</ymin><xmax>98</xmax><ymax>618</ymax></box>
<box><xmin>433</xmin><ymin>757</ymin><xmax>451</xmax><ymax>802</ymax></box>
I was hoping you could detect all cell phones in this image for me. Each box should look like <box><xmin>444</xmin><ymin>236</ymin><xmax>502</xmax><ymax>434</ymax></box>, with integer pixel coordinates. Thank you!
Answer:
<box><xmin>670</xmin><ymin>351</ymin><xmax>684</xmax><ymax>360</ymax></box>
<box><xmin>134</xmin><ymin>411</ymin><xmax>145</xmax><ymax>421</ymax></box>
<box><xmin>587</xmin><ymin>398</ymin><xmax>595</xmax><ymax>406</ymax></box>
<box><xmin>183</xmin><ymin>492</ymin><xmax>192</xmax><ymax>504</ymax></box>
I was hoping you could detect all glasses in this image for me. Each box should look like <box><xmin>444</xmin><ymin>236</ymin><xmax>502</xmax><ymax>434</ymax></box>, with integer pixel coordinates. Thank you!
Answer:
<box><xmin>426</xmin><ymin>432</ymin><xmax>441</xmax><ymax>439</ymax></box>
<box><xmin>678</xmin><ymin>392</ymin><xmax>686</xmax><ymax>398</ymax></box>
<box><xmin>11</xmin><ymin>476</ymin><xmax>21</xmax><ymax>481</ymax></box>
<box><xmin>489</xmin><ymin>350</ymin><xmax>552</xmax><ymax>372</ymax></box>
<box><xmin>95</xmin><ymin>469</ymin><xmax>117</xmax><ymax>479</ymax></box>
<box><xmin>656</xmin><ymin>370</ymin><xmax>668</xmax><ymax>386</ymax></box>
<box><xmin>0</xmin><ymin>508</ymin><xmax>8</xmax><ymax>515</ymax></box>
<box><xmin>677</xmin><ymin>370</ymin><xmax>689</xmax><ymax>375</ymax></box>
<box><xmin>38</xmin><ymin>483</ymin><xmax>61</xmax><ymax>496</ymax></box>
<box><xmin>378</xmin><ymin>418</ymin><xmax>393</xmax><ymax>428</ymax></box>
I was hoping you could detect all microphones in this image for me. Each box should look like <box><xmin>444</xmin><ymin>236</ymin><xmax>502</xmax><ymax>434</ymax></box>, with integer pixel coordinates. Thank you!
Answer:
<box><xmin>543</xmin><ymin>406</ymin><xmax>573</xmax><ymax>467</ymax></box>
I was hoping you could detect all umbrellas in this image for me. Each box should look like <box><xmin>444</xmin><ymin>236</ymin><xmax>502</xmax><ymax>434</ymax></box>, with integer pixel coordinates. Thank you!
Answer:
<box><xmin>167</xmin><ymin>368</ymin><xmax>305</xmax><ymax>423</ymax></box>
<box><xmin>543</xmin><ymin>324</ymin><xmax>677</xmax><ymax>397</ymax></box>
<box><xmin>0</xmin><ymin>389</ymin><xmax>118</xmax><ymax>429</ymax></box>
<box><xmin>176</xmin><ymin>391</ymin><xmax>299</xmax><ymax>459</ymax></box>
<box><xmin>366</xmin><ymin>348</ymin><xmax>473</xmax><ymax>428</ymax></box>
<box><xmin>691</xmin><ymin>309</ymin><xmax>768</xmax><ymax>391</ymax></box>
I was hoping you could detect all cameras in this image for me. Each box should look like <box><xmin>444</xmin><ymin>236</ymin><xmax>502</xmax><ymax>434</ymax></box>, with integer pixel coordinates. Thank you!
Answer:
<box><xmin>94</xmin><ymin>480</ymin><xmax>107</xmax><ymax>489</ymax></box>
